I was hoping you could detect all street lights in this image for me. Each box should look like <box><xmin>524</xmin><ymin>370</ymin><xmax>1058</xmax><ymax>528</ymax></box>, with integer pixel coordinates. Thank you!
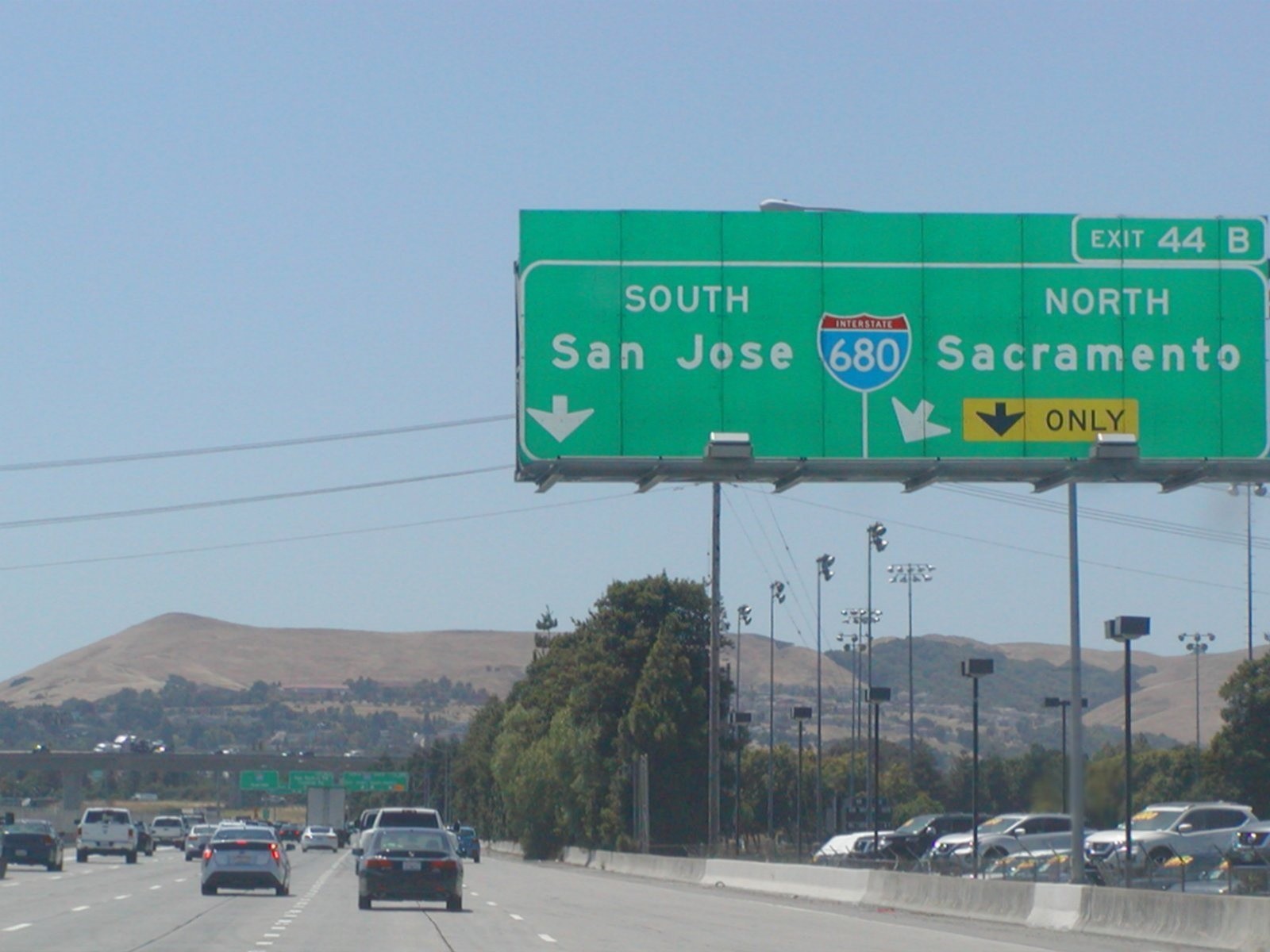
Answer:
<box><xmin>734</xmin><ymin>520</ymin><xmax>1270</xmax><ymax>887</ymax></box>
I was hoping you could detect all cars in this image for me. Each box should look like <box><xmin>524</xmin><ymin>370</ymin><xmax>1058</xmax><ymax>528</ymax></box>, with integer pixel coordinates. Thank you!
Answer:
<box><xmin>73</xmin><ymin>807</ymin><xmax>140</xmax><ymax>865</ymax></box>
<box><xmin>2</xmin><ymin>817</ymin><xmax>64</xmax><ymax>872</ymax></box>
<box><xmin>200</xmin><ymin>825</ymin><xmax>290</xmax><ymax>898</ymax></box>
<box><xmin>352</xmin><ymin>827</ymin><xmax>467</xmax><ymax>912</ymax></box>
<box><xmin>132</xmin><ymin>806</ymin><xmax>483</xmax><ymax>863</ymax></box>
<box><xmin>810</xmin><ymin>799</ymin><xmax>1269</xmax><ymax>900</ymax></box>
<box><xmin>25</xmin><ymin>734</ymin><xmax>375</xmax><ymax>760</ymax></box>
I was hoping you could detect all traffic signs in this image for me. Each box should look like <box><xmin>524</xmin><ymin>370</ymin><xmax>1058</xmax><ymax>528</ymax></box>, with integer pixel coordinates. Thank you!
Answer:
<box><xmin>514</xmin><ymin>200</ymin><xmax>1270</xmax><ymax>494</ymax></box>
<box><xmin>238</xmin><ymin>769</ymin><xmax>410</xmax><ymax>792</ymax></box>
